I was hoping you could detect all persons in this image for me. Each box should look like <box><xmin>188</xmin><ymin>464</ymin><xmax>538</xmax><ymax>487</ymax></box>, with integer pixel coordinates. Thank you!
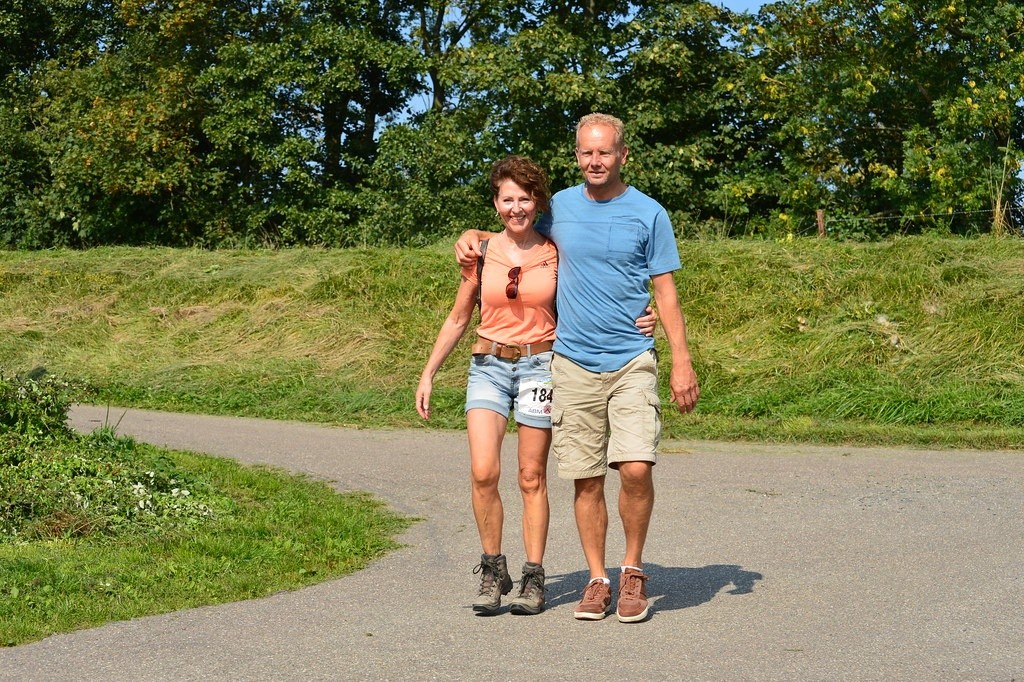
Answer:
<box><xmin>414</xmin><ymin>155</ymin><xmax>658</xmax><ymax>615</ymax></box>
<box><xmin>456</xmin><ymin>114</ymin><xmax>700</xmax><ymax>623</ymax></box>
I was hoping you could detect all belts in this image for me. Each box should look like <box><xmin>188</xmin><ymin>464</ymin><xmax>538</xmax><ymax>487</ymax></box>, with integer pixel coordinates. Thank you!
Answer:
<box><xmin>472</xmin><ymin>336</ymin><xmax>553</xmax><ymax>360</ymax></box>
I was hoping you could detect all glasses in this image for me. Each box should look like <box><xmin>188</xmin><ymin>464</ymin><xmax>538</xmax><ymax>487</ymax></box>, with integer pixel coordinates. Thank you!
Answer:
<box><xmin>506</xmin><ymin>267</ymin><xmax>521</xmax><ymax>299</ymax></box>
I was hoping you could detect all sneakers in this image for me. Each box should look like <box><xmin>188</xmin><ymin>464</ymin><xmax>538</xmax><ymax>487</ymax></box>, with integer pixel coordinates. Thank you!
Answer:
<box><xmin>510</xmin><ymin>562</ymin><xmax>548</xmax><ymax>614</ymax></box>
<box><xmin>574</xmin><ymin>577</ymin><xmax>611</xmax><ymax>620</ymax></box>
<box><xmin>472</xmin><ymin>554</ymin><xmax>513</xmax><ymax>615</ymax></box>
<box><xmin>617</xmin><ymin>565</ymin><xmax>648</xmax><ymax>623</ymax></box>
